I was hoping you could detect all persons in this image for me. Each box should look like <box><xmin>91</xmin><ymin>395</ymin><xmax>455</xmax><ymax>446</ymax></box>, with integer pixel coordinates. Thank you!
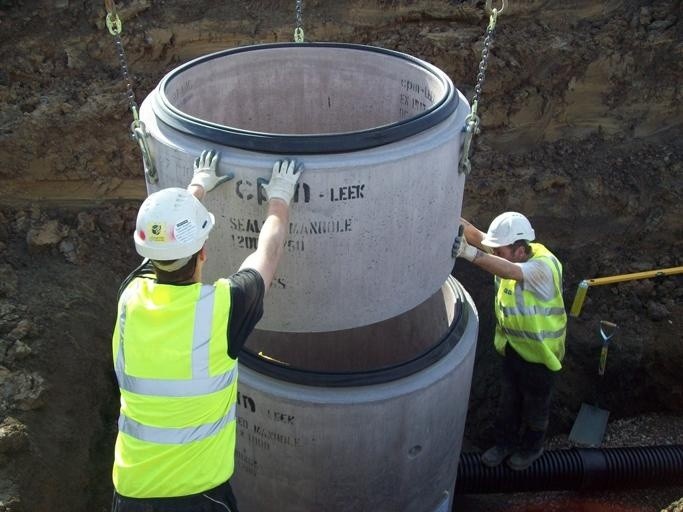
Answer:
<box><xmin>448</xmin><ymin>209</ymin><xmax>570</xmax><ymax>476</ymax></box>
<box><xmin>109</xmin><ymin>148</ymin><xmax>306</xmax><ymax>510</ymax></box>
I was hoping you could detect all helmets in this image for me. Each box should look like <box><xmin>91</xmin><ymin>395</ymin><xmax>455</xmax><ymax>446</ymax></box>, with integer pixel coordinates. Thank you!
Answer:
<box><xmin>480</xmin><ymin>210</ymin><xmax>535</xmax><ymax>249</ymax></box>
<box><xmin>133</xmin><ymin>187</ymin><xmax>217</xmax><ymax>261</ymax></box>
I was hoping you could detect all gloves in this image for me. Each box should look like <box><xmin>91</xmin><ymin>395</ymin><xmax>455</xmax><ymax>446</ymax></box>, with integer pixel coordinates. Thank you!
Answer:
<box><xmin>188</xmin><ymin>148</ymin><xmax>235</xmax><ymax>196</ymax></box>
<box><xmin>450</xmin><ymin>224</ymin><xmax>478</xmax><ymax>264</ymax></box>
<box><xmin>259</xmin><ymin>157</ymin><xmax>307</xmax><ymax>206</ymax></box>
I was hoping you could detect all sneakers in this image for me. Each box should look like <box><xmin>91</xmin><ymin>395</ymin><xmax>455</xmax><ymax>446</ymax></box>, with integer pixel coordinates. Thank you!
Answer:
<box><xmin>482</xmin><ymin>443</ymin><xmax>509</xmax><ymax>468</ymax></box>
<box><xmin>507</xmin><ymin>444</ymin><xmax>544</xmax><ymax>472</ymax></box>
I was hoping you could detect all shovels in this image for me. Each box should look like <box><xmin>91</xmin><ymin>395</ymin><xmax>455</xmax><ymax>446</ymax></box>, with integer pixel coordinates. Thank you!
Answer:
<box><xmin>568</xmin><ymin>320</ymin><xmax>618</xmax><ymax>448</ymax></box>
